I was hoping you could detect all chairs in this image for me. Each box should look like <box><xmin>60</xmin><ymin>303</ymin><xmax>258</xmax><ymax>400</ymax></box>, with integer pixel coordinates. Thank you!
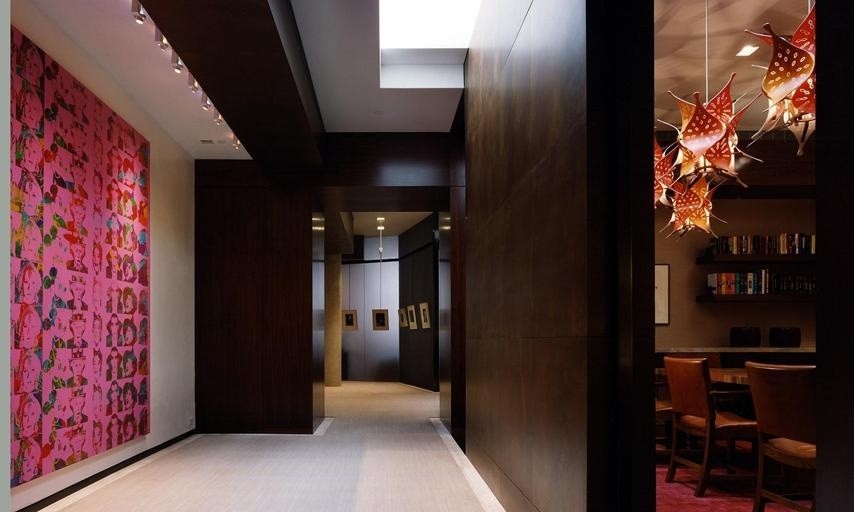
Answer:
<box><xmin>656</xmin><ymin>353</ymin><xmax>817</xmax><ymax>512</ymax></box>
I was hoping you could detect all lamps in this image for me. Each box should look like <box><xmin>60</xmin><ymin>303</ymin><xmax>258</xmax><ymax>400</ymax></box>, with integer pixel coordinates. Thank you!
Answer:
<box><xmin>656</xmin><ymin>2</ymin><xmax>817</xmax><ymax>241</ymax></box>
<box><xmin>130</xmin><ymin>0</ymin><xmax>224</xmax><ymax>128</ymax></box>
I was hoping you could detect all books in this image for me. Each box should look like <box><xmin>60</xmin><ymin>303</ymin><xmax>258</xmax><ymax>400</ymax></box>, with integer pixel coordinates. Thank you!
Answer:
<box><xmin>706</xmin><ymin>231</ymin><xmax>820</xmax><ymax>295</ymax></box>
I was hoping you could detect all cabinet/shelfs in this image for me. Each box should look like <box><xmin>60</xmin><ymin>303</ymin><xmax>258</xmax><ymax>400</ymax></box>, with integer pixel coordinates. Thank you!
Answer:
<box><xmin>695</xmin><ymin>251</ymin><xmax>818</xmax><ymax>306</ymax></box>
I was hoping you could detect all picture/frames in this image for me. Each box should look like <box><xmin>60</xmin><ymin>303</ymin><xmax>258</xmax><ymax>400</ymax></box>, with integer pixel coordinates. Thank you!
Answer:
<box><xmin>342</xmin><ymin>301</ymin><xmax>433</xmax><ymax>332</ymax></box>
<box><xmin>655</xmin><ymin>263</ymin><xmax>671</xmax><ymax>327</ymax></box>
